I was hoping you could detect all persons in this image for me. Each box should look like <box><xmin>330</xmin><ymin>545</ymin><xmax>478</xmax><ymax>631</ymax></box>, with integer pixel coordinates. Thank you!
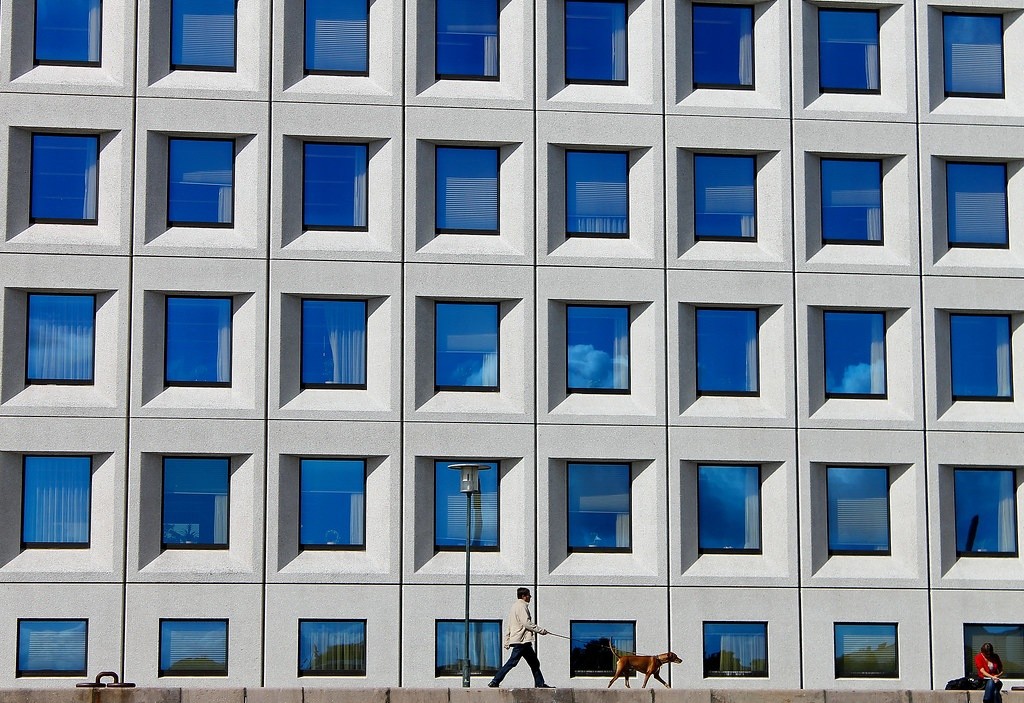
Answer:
<box><xmin>489</xmin><ymin>588</ymin><xmax>549</xmax><ymax>688</ymax></box>
<box><xmin>975</xmin><ymin>643</ymin><xmax>1004</xmax><ymax>703</ymax></box>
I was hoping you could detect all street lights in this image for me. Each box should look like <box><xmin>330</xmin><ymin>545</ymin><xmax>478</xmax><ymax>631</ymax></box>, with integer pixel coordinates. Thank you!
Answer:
<box><xmin>447</xmin><ymin>463</ymin><xmax>491</xmax><ymax>690</ymax></box>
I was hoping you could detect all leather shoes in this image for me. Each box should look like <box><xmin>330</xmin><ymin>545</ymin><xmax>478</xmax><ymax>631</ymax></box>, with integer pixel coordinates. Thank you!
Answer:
<box><xmin>488</xmin><ymin>682</ymin><xmax>499</xmax><ymax>687</ymax></box>
<box><xmin>535</xmin><ymin>684</ymin><xmax>556</xmax><ymax>688</ymax></box>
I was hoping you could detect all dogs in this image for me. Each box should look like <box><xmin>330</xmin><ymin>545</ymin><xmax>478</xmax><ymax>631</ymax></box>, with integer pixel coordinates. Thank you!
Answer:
<box><xmin>607</xmin><ymin>636</ymin><xmax>682</xmax><ymax>688</ymax></box>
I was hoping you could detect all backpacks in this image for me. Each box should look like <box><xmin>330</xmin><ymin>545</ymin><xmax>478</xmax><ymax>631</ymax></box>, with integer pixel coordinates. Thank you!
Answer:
<box><xmin>945</xmin><ymin>677</ymin><xmax>985</xmax><ymax>690</ymax></box>
